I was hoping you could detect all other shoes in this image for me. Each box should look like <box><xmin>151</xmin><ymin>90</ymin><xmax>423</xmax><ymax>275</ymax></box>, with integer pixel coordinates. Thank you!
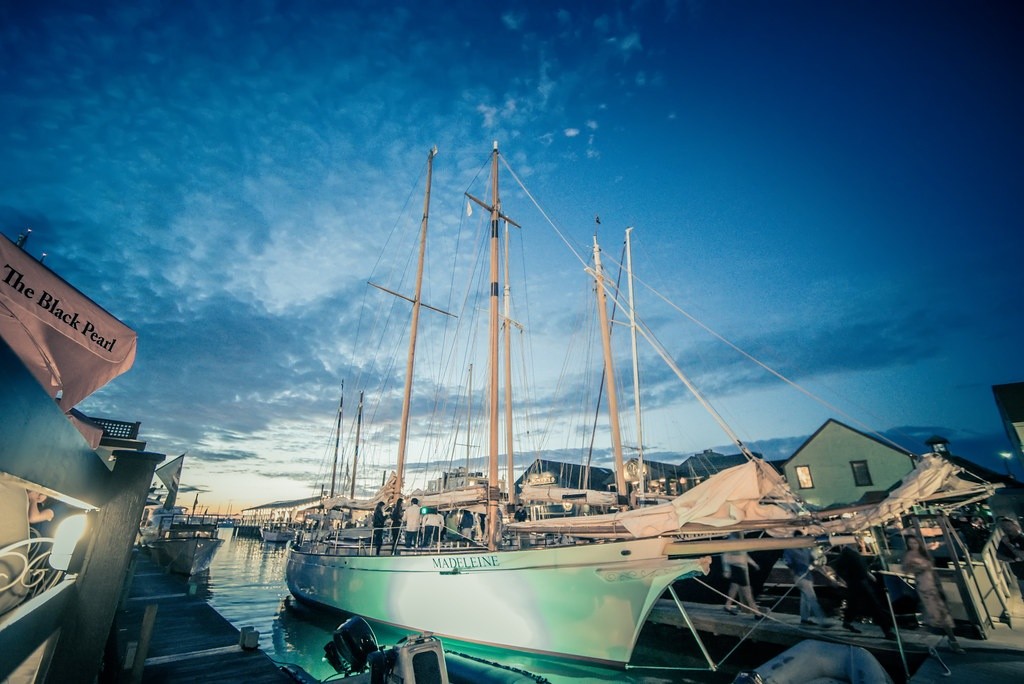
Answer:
<box><xmin>800</xmin><ymin>620</ymin><xmax>817</xmax><ymax>629</ymax></box>
<box><xmin>885</xmin><ymin>632</ymin><xmax>901</xmax><ymax>641</ymax></box>
<box><xmin>843</xmin><ymin>624</ymin><xmax>862</xmax><ymax>634</ymax></box>
<box><xmin>820</xmin><ymin>622</ymin><xmax>835</xmax><ymax>628</ymax></box>
<box><xmin>755</xmin><ymin>614</ymin><xmax>768</xmax><ymax>620</ymax></box>
<box><xmin>723</xmin><ymin>606</ymin><xmax>738</xmax><ymax>615</ymax></box>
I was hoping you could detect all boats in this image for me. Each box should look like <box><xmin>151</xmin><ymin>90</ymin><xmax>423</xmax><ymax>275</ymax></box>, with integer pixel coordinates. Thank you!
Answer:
<box><xmin>0</xmin><ymin>138</ymin><xmax>1024</xmax><ymax>683</ymax></box>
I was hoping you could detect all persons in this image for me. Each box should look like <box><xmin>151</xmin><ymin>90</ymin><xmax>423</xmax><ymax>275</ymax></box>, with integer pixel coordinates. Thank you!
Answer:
<box><xmin>827</xmin><ymin>533</ymin><xmax>901</xmax><ymax>641</ymax></box>
<box><xmin>513</xmin><ymin>503</ymin><xmax>528</xmax><ymax>523</ymax></box>
<box><xmin>965</xmin><ymin>516</ymin><xmax>989</xmax><ymax>553</ymax></box>
<box><xmin>721</xmin><ymin>531</ymin><xmax>770</xmax><ymax>621</ymax></box>
<box><xmin>781</xmin><ymin>530</ymin><xmax>836</xmax><ymax>629</ymax></box>
<box><xmin>373</xmin><ymin>498</ymin><xmax>503</xmax><ymax>555</ymax></box>
<box><xmin>28</xmin><ymin>491</ymin><xmax>75</xmax><ymax>590</ymax></box>
<box><xmin>996</xmin><ymin>517</ymin><xmax>1024</xmax><ymax>601</ymax></box>
<box><xmin>901</xmin><ymin>534</ymin><xmax>967</xmax><ymax>654</ymax></box>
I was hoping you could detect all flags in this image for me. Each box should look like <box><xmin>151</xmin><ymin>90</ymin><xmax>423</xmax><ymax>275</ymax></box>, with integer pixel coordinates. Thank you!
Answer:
<box><xmin>155</xmin><ymin>453</ymin><xmax>184</xmax><ymax>511</ymax></box>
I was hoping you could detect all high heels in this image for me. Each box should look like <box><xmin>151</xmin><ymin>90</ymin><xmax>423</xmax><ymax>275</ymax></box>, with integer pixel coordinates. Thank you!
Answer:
<box><xmin>949</xmin><ymin>640</ymin><xmax>966</xmax><ymax>654</ymax></box>
